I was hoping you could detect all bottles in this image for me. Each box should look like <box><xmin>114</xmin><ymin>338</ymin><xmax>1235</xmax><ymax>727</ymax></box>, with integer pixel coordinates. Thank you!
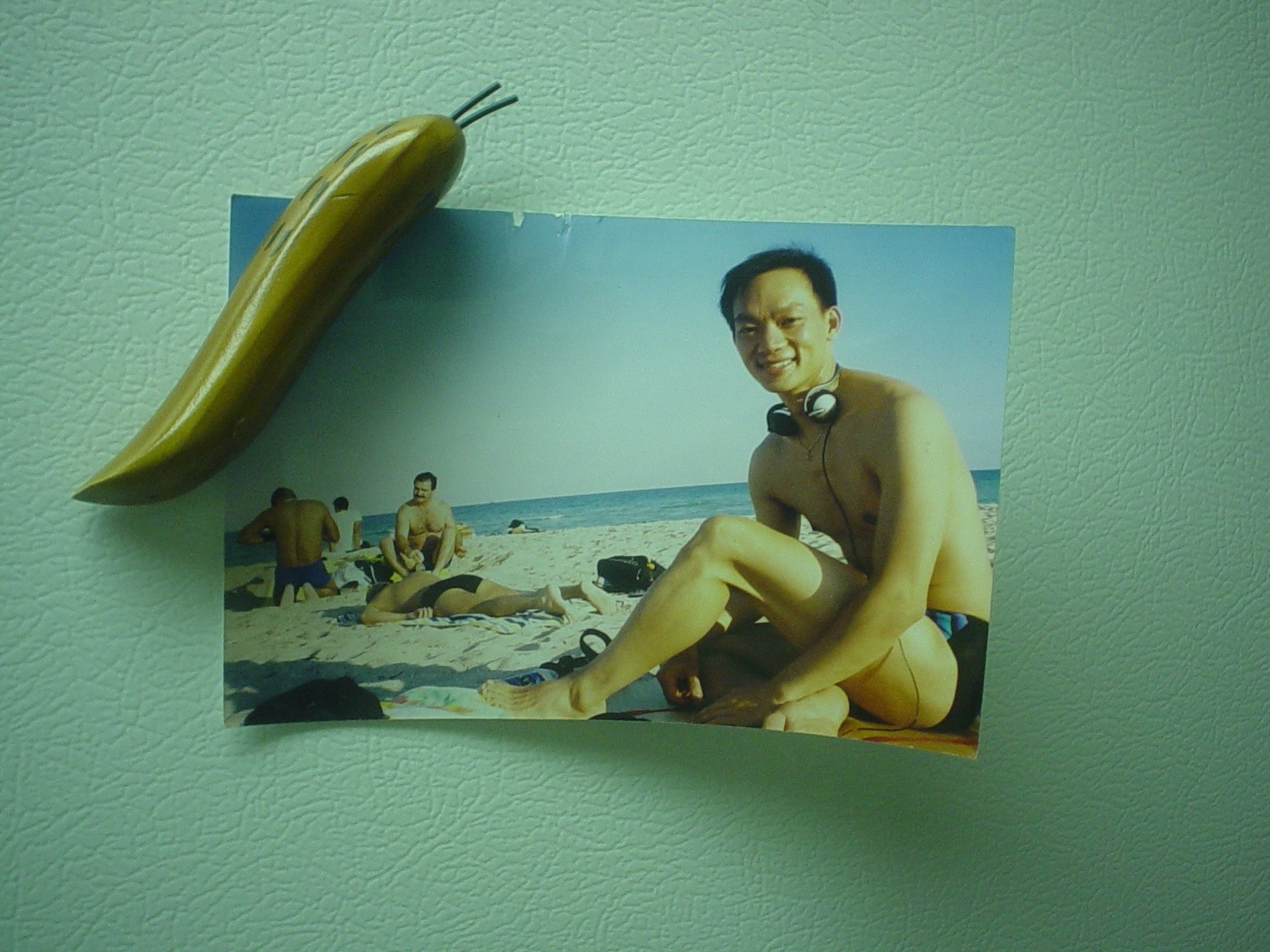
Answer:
<box><xmin>497</xmin><ymin>668</ymin><xmax>558</xmax><ymax>688</ymax></box>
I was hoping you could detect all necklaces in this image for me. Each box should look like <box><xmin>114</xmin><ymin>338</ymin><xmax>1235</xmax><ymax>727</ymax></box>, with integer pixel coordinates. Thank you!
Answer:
<box><xmin>795</xmin><ymin>421</ymin><xmax>827</xmax><ymax>461</ymax></box>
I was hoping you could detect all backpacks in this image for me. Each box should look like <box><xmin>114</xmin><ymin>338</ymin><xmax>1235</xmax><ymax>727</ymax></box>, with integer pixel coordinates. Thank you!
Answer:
<box><xmin>592</xmin><ymin>554</ymin><xmax>665</xmax><ymax>596</ymax></box>
<box><xmin>240</xmin><ymin>676</ymin><xmax>388</xmax><ymax>727</ymax></box>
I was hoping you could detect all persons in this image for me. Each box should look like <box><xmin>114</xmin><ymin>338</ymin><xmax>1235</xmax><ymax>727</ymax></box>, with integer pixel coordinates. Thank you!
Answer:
<box><xmin>361</xmin><ymin>570</ymin><xmax>622</xmax><ymax>625</ymax></box>
<box><xmin>477</xmin><ymin>240</ymin><xmax>995</xmax><ymax>739</ymax></box>
<box><xmin>377</xmin><ymin>471</ymin><xmax>468</xmax><ymax>578</ymax></box>
<box><xmin>323</xmin><ymin>495</ymin><xmax>366</xmax><ymax>555</ymax></box>
<box><xmin>236</xmin><ymin>486</ymin><xmax>341</xmax><ymax>608</ymax></box>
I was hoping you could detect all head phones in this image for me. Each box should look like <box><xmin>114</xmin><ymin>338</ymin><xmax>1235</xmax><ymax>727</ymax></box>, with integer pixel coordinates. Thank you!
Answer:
<box><xmin>764</xmin><ymin>365</ymin><xmax>841</xmax><ymax>438</ymax></box>
